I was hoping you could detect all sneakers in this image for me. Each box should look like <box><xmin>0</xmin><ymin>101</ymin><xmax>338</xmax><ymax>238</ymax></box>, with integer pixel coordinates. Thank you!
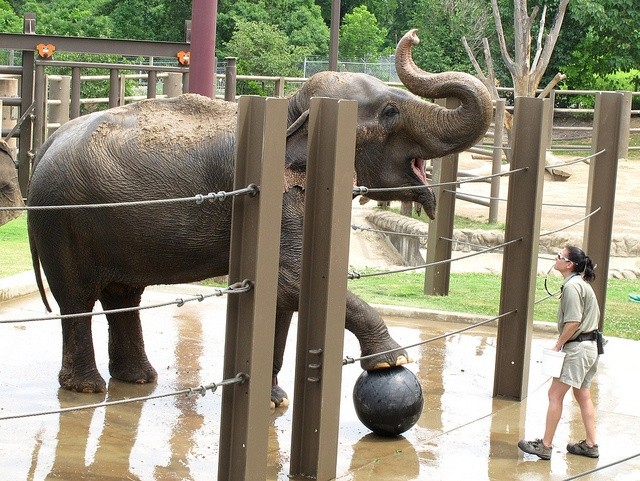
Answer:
<box><xmin>567</xmin><ymin>439</ymin><xmax>599</xmax><ymax>458</ymax></box>
<box><xmin>518</xmin><ymin>438</ymin><xmax>552</xmax><ymax>460</ymax></box>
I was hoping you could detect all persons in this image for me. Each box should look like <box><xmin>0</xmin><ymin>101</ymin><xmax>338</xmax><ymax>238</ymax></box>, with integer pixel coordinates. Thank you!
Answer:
<box><xmin>517</xmin><ymin>247</ymin><xmax>606</xmax><ymax>461</ymax></box>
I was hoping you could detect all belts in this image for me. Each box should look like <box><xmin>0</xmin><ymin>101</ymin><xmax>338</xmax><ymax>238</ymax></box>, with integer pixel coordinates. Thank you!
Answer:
<box><xmin>566</xmin><ymin>331</ymin><xmax>599</xmax><ymax>342</ymax></box>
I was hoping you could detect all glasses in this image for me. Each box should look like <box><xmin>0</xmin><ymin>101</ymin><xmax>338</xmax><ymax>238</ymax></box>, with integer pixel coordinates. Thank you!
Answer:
<box><xmin>557</xmin><ymin>251</ymin><xmax>578</xmax><ymax>265</ymax></box>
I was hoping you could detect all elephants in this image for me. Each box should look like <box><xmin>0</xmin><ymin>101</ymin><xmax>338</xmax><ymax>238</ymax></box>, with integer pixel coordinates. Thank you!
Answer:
<box><xmin>0</xmin><ymin>137</ymin><xmax>26</xmax><ymax>226</ymax></box>
<box><xmin>26</xmin><ymin>27</ymin><xmax>493</xmax><ymax>409</ymax></box>
